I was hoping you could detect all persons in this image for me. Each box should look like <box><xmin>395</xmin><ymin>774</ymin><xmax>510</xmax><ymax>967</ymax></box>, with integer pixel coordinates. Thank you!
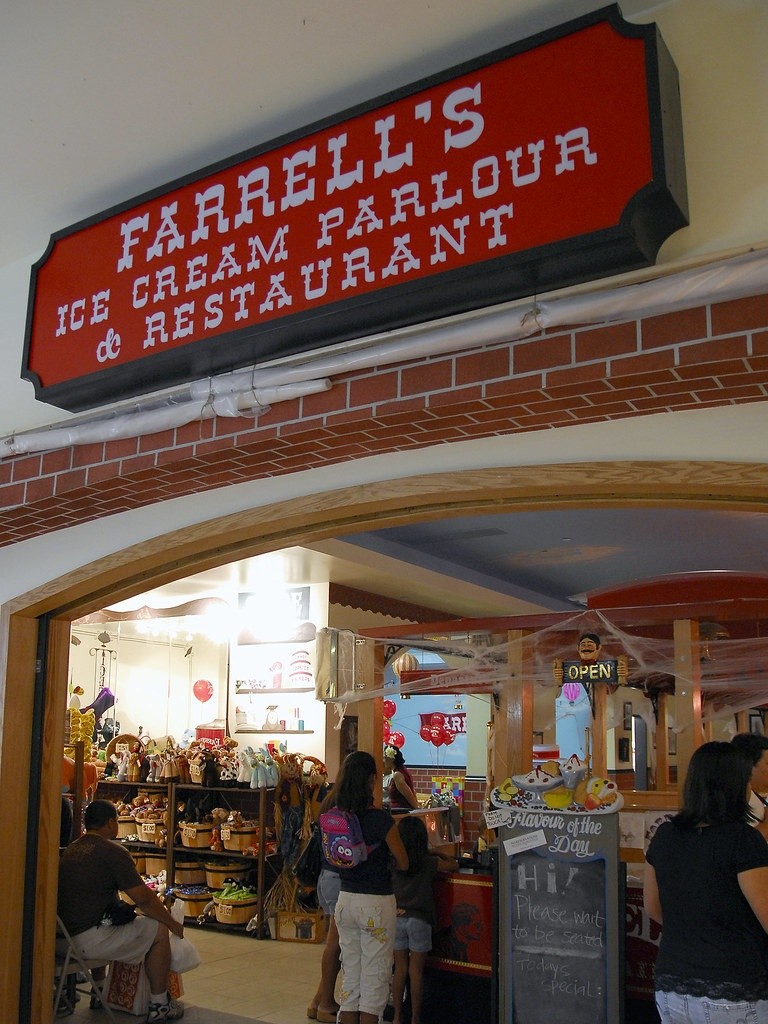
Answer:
<box><xmin>382</xmin><ymin>745</ymin><xmax>422</xmax><ymax>811</ymax></box>
<box><xmin>334</xmin><ymin>750</ymin><xmax>410</xmax><ymax>1024</ymax></box>
<box><xmin>391</xmin><ymin>816</ymin><xmax>442</xmax><ymax>1024</ymax></box>
<box><xmin>731</xmin><ymin>733</ymin><xmax>768</xmax><ymax>844</ymax></box>
<box><xmin>304</xmin><ymin>764</ymin><xmax>331</xmax><ymax>828</ymax></box>
<box><xmin>307</xmin><ymin>793</ymin><xmax>341</xmax><ymax>1022</ymax></box>
<box><xmin>644</xmin><ymin>741</ymin><xmax>768</xmax><ymax>1024</ymax></box>
<box><xmin>274</xmin><ymin>754</ymin><xmax>306</xmax><ymax>865</ymax></box>
<box><xmin>55</xmin><ymin>800</ymin><xmax>185</xmax><ymax>1024</ymax></box>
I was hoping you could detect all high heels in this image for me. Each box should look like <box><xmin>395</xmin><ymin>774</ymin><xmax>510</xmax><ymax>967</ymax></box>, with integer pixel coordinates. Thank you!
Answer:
<box><xmin>307</xmin><ymin>1008</ymin><xmax>317</xmax><ymax>1018</ymax></box>
<box><xmin>317</xmin><ymin>1005</ymin><xmax>341</xmax><ymax>1022</ymax></box>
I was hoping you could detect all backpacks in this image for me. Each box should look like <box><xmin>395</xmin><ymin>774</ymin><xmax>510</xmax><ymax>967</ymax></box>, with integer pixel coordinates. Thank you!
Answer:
<box><xmin>320</xmin><ymin>806</ymin><xmax>382</xmax><ymax>868</ymax></box>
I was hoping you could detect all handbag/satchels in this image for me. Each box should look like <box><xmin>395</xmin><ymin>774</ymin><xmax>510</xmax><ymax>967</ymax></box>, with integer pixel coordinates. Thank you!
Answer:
<box><xmin>292</xmin><ymin>828</ymin><xmax>321</xmax><ymax>887</ymax></box>
<box><xmin>169</xmin><ymin>899</ymin><xmax>202</xmax><ymax>975</ymax></box>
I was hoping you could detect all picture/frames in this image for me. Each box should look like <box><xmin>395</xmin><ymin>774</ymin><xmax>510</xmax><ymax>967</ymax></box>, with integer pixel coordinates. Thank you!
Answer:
<box><xmin>749</xmin><ymin>713</ymin><xmax>764</xmax><ymax>734</ymax></box>
<box><xmin>623</xmin><ymin>702</ymin><xmax>634</xmax><ymax>730</ymax></box>
<box><xmin>668</xmin><ymin>727</ymin><xmax>677</xmax><ymax>755</ymax></box>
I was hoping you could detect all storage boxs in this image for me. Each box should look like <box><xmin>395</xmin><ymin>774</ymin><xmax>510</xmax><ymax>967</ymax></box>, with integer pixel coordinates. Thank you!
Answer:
<box><xmin>277</xmin><ymin>908</ymin><xmax>327</xmax><ymax>944</ymax></box>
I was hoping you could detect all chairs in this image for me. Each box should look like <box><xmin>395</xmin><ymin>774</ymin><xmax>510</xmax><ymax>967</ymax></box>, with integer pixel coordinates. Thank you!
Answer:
<box><xmin>53</xmin><ymin>916</ymin><xmax>118</xmax><ymax>1024</ymax></box>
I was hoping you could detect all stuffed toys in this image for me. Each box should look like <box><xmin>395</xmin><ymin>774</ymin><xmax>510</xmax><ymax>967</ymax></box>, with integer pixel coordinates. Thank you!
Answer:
<box><xmin>104</xmin><ymin>794</ymin><xmax>169</xmax><ymax>847</ymax></box>
<box><xmin>174</xmin><ymin>801</ymin><xmax>281</xmax><ymax>923</ymax></box>
<box><xmin>62</xmin><ymin>757</ymin><xmax>96</xmax><ymax>802</ymax></box>
<box><xmin>90</xmin><ymin>737</ymin><xmax>279</xmax><ymax>789</ymax></box>
<box><xmin>140</xmin><ymin>870</ymin><xmax>167</xmax><ymax>898</ymax></box>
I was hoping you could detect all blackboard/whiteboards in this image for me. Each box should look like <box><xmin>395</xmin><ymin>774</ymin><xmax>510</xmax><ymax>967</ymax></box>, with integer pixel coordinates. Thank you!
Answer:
<box><xmin>499</xmin><ymin>814</ymin><xmax>619</xmax><ymax>1023</ymax></box>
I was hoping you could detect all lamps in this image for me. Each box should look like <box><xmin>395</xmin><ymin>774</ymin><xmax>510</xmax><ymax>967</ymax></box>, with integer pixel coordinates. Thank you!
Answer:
<box><xmin>698</xmin><ymin>621</ymin><xmax>731</xmax><ymax>661</ymax></box>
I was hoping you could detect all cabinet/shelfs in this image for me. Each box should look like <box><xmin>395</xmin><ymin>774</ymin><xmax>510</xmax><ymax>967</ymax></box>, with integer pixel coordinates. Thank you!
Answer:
<box><xmin>97</xmin><ymin>780</ymin><xmax>283</xmax><ymax>940</ymax></box>
<box><xmin>234</xmin><ymin>687</ymin><xmax>315</xmax><ymax>734</ymax></box>
<box><xmin>58</xmin><ymin>741</ymin><xmax>85</xmax><ymax>849</ymax></box>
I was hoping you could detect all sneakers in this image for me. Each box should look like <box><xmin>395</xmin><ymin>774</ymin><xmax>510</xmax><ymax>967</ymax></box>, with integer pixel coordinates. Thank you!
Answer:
<box><xmin>146</xmin><ymin>991</ymin><xmax>184</xmax><ymax>1024</ymax></box>
<box><xmin>90</xmin><ymin>987</ymin><xmax>103</xmax><ymax>1009</ymax></box>
